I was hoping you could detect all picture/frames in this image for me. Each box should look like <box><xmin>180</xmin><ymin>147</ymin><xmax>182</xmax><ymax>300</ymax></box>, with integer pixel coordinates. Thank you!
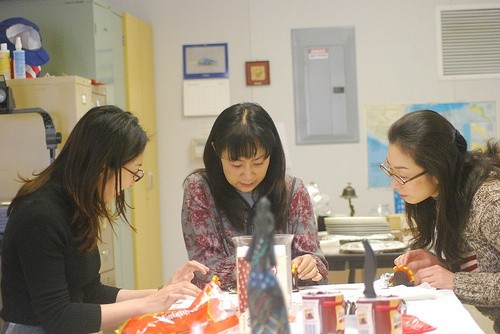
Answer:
<box><xmin>246</xmin><ymin>61</ymin><xmax>270</xmax><ymax>85</ymax></box>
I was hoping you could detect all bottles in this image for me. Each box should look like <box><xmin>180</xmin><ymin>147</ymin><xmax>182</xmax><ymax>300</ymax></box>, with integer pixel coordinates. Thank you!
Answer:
<box><xmin>13</xmin><ymin>37</ymin><xmax>26</xmax><ymax>79</ymax></box>
<box><xmin>0</xmin><ymin>43</ymin><xmax>10</xmax><ymax>80</ymax></box>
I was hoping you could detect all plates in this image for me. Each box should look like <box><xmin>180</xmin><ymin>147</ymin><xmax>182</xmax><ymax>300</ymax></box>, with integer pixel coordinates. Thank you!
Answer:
<box><xmin>340</xmin><ymin>242</ymin><xmax>406</xmax><ymax>253</ymax></box>
<box><xmin>324</xmin><ymin>217</ymin><xmax>391</xmax><ymax>236</ymax></box>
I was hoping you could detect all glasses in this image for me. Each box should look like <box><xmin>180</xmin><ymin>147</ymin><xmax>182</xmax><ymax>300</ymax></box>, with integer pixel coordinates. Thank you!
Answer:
<box><xmin>379</xmin><ymin>160</ymin><xmax>430</xmax><ymax>185</ymax></box>
<box><xmin>121</xmin><ymin>166</ymin><xmax>145</xmax><ymax>182</ymax></box>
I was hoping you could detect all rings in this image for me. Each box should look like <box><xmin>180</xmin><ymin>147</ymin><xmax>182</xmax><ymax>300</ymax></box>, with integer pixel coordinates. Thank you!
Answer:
<box><xmin>314</xmin><ymin>267</ymin><xmax>320</xmax><ymax>273</ymax></box>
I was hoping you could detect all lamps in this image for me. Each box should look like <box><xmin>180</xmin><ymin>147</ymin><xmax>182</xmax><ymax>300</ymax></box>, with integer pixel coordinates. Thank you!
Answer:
<box><xmin>339</xmin><ymin>183</ymin><xmax>359</xmax><ymax>217</ymax></box>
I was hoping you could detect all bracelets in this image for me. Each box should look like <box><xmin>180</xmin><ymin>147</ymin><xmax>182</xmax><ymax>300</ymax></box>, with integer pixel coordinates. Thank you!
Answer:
<box><xmin>158</xmin><ymin>285</ymin><xmax>164</xmax><ymax>290</ymax></box>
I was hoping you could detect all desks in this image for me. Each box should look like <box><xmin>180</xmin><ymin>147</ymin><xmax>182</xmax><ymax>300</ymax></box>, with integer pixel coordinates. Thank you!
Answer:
<box><xmin>318</xmin><ymin>230</ymin><xmax>446</xmax><ymax>284</ymax></box>
<box><xmin>167</xmin><ymin>282</ymin><xmax>487</xmax><ymax>334</ymax></box>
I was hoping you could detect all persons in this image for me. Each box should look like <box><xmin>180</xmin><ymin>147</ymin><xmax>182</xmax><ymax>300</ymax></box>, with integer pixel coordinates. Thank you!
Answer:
<box><xmin>0</xmin><ymin>105</ymin><xmax>209</xmax><ymax>334</ymax></box>
<box><xmin>181</xmin><ymin>103</ymin><xmax>329</xmax><ymax>292</ymax></box>
<box><xmin>379</xmin><ymin>110</ymin><xmax>500</xmax><ymax>334</ymax></box>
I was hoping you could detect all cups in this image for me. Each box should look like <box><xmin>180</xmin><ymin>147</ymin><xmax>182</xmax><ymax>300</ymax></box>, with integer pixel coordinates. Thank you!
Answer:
<box><xmin>232</xmin><ymin>234</ymin><xmax>295</xmax><ymax>327</ymax></box>
